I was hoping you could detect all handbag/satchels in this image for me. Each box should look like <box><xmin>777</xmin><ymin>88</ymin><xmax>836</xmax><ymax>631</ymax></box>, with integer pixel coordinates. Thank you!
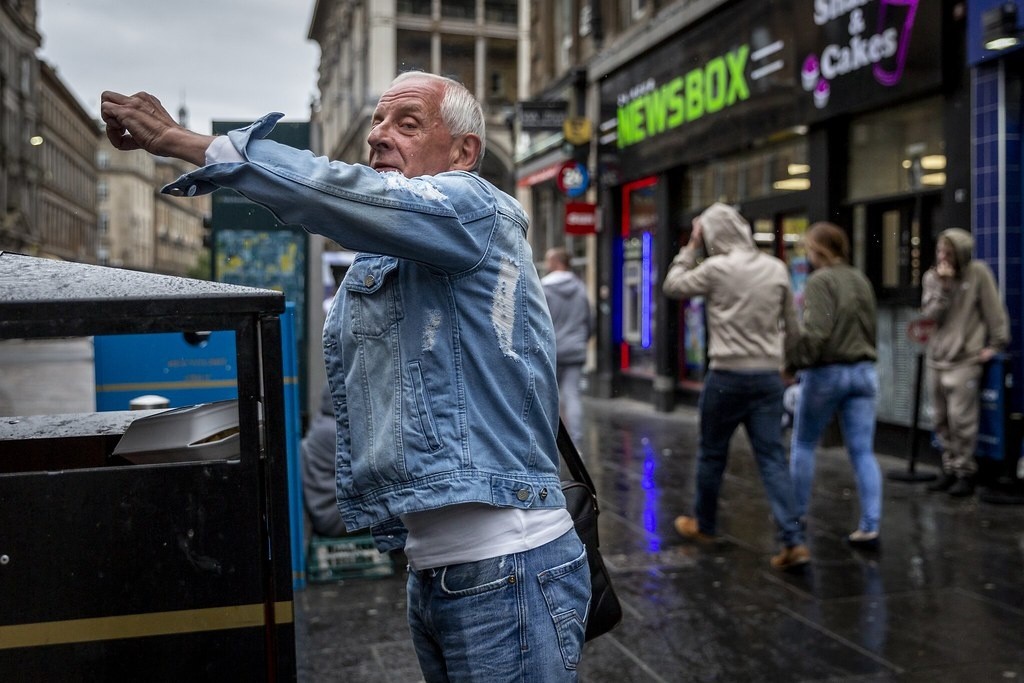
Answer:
<box><xmin>557</xmin><ymin>415</ymin><xmax>624</xmax><ymax>644</ymax></box>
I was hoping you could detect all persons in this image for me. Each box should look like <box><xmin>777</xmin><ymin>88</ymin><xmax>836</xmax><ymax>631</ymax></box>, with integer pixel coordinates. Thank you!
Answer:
<box><xmin>100</xmin><ymin>68</ymin><xmax>593</xmax><ymax>683</ymax></box>
<box><xmin>540</xmin><ymin>246</ymin><xmax>596</xmax><ymax>456</ymax></box>
<box><xmin>920</xmin><ymin>228</ymin><xmax>1012</xmax><ymax>497</ymax></box>
<box><xmin>664</xmin><ymin>203</ymin><xmax>812</xmax><ymax>568</ymax></box>
<box><xmin>302</xmin><ymin>382</ymin><xmax>371</xmax><ymax>538</ymax></box>
<box><xmin>788</xmin><ymin>222</ymin><xmax>882</xmax><ymax>546</ymax></box>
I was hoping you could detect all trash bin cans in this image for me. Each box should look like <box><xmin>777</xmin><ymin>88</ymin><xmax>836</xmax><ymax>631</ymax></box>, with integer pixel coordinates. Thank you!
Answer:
<box><xmin>1</xmin><ymin>251</ymin><xmax>302</xmax><ymax>677</ymax></box>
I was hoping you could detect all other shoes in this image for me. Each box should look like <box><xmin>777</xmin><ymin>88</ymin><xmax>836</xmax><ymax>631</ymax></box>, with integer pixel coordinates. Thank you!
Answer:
<box><xmin>676</xmin><ymin>516</ymin><xmax>717</xmax><ymax>544</ymax></box>
<box><xmin>767</xmin><ymin>511</ymin><xmax>808</xmax><ymax>529</ymax></box>
<box><xmin>940</xmin><ymin>474</ymin><xmax>977</xmax><ymax>497</ymax></box>
<box><xmin>844</xmin><ymin>529</ymin><xmax>882</xmax><ymax>549</ymax></box>
<box><xmin>769</xmin><ymin>544</ymin><xmax>811</xmax><ymax>567</ymax></box>
